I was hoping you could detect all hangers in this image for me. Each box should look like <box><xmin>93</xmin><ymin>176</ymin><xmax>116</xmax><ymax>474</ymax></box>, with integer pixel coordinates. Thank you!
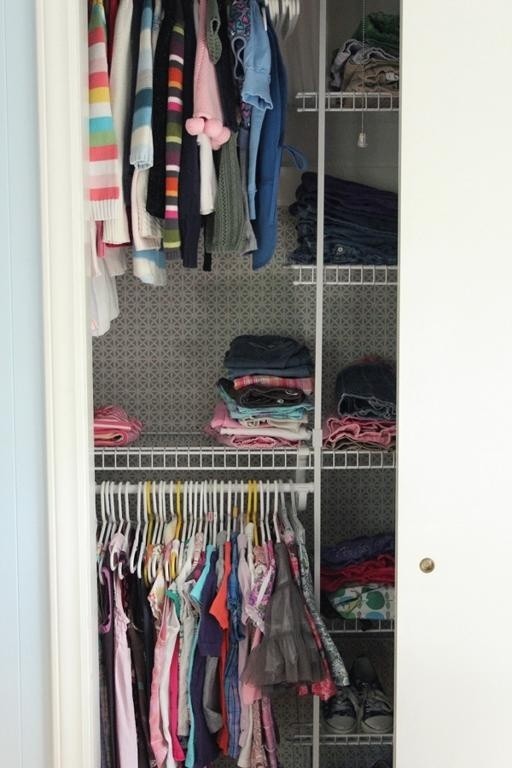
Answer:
<box><xmin>95</xmin><ymin>479</ymin><xmax>306</xmax><ymax>587</ymax></box>
<box><xmin>95</xmin><ymin>427</ymin><xmax>323</xmax><ymax>768</ymax></box>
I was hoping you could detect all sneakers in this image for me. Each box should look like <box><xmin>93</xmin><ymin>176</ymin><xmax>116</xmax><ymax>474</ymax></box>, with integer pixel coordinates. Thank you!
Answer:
<box><xmin>348</xmin><ymin>654</ymin><xmax>393</xmax><ymax>734</ymax></box>
<box><xmin>320</xmin><ymin>684</ymin><xmax>357</xmax><ymax>736</ymax></box>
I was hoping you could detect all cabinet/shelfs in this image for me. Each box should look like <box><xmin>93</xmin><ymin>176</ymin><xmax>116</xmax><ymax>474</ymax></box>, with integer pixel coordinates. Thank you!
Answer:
<box><xmin>1</xmin><ymin>0</ymin><xmax>512</xmax><ymax>768</ymax></box>
